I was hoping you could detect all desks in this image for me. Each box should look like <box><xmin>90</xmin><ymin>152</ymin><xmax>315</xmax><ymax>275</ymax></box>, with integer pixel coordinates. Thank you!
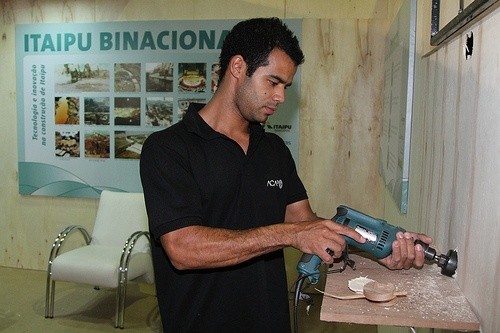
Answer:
<box><xmin>320</xmin><ymin>248</ymin><xmax>481</xmax><ymax>333</ymax></box>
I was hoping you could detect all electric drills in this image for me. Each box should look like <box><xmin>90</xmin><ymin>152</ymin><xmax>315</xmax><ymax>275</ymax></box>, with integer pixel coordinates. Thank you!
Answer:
<box><xmin>296</xmin><ymin>205</ymin><xmax>458</xmax><ymax>285</ymax></box>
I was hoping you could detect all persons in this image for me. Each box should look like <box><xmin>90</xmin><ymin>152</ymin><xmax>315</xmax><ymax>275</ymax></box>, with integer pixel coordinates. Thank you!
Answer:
<box><xmin>140</xmin><ymin>17</ymin><xmax>432</xmax><ymax>333</ymax></box>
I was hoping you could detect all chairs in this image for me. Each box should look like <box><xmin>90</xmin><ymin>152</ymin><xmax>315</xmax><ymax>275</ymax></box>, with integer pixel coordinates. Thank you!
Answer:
<box><xmin>44</xmin><ymin>190</ymin><xmax>154</xmax><ymax>329</ymax></box>
